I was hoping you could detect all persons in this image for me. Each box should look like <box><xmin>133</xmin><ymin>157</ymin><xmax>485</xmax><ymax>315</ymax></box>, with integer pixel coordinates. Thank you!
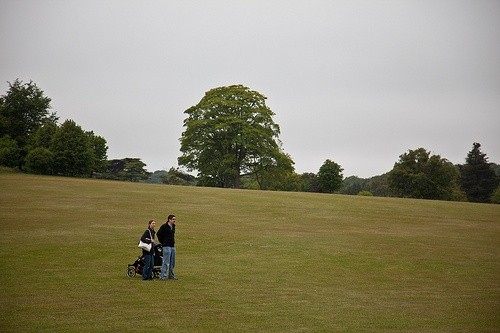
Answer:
<box><xmin>156</xmin><ymin>214</ymin><xmax>178</xmax><ymax>281</ymax></box>
<box><xmin>141</xmin><ymin>219</ymin><xmax>159</xmax><ymax>280</ymax></box>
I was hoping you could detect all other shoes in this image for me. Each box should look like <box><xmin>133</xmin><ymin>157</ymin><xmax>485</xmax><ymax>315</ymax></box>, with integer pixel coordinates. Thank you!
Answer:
<box><xmin>160</xmin><ymin>276</ymin><xmax>167</xmax><ymax>281</ymax></box>
<box><xmin>143</xmin><ymin>277</ymin><xmax>152</xmax><ymax>280</ymax></box>
<box><xmin>169</xmin><ymin>277</ymin><xmax>178</xmax><ymax>280</ymax></box>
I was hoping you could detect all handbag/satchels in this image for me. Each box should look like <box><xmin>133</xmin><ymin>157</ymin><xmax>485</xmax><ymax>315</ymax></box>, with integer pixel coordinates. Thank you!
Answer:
<box><xmin>138</xmin><ymin>229</ymin><xmax>152</xmax><ymax>252</ymax></box>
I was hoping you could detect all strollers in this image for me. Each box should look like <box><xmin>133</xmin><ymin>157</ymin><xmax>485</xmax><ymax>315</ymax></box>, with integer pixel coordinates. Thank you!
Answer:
<box><xmin>128</xmin><ymin>244</ymin><xmax>163</xmax><ymax>277</ymax></box>
